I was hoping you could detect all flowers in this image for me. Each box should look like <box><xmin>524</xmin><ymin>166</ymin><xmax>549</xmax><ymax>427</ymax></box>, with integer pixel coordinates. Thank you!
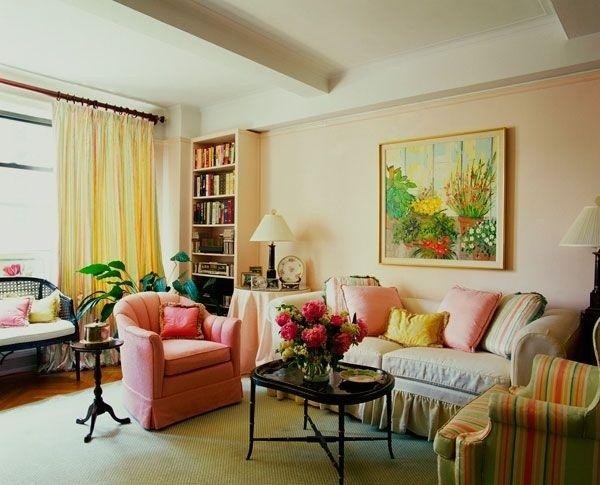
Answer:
<box><xmin>409</xmin><ymin>161</ymin><xmax>497</xmax><ymax>260</ymax></box>
<box><xmin>270</xmin><ymin>299</ymin><xmax>370</xmax><ymax>391</ymax></box>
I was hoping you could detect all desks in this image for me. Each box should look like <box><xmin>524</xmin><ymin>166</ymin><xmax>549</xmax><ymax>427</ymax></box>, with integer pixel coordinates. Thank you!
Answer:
<box><xmin>230</xmin><ymin>285</ymin><xmax>312</xmax><ymax>378</ymax></box>
<box><xmin>69</xmin><ymin>337</ymin><xmax>132</xmax><ymax>445</ymax></box>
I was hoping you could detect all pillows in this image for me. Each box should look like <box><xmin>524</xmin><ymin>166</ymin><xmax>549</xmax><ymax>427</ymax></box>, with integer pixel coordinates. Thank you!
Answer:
<box><xmin>0</xmin><ymin>295</ymin><xmax>36</xmax><ymax>328</ymax></box>
<box><xmin>157</xmin><ymin>301</ymin><xmax>206</xmax><ymax>342</ymax></box>
<box><xmin>28</xmin><ymin>289</ymin><xmax>62</xmax><ymax>325</ymax></box>
<box><xmin>324</xmin><ymin>275</ymin><xmax>550</xmax><ymax>361</ymax></box>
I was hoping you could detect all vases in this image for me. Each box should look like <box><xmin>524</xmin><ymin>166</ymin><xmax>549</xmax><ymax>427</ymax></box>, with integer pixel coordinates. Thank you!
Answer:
<box><xmin>301</xmin><ymin>366</ymin><xmax>332</xmax><ymax>391</ymax></box>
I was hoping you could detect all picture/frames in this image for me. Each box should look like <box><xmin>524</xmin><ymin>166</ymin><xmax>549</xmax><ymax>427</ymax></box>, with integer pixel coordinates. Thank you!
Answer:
<box><xmin>240</xmin><ymin>271</ymin><xmax>262</xmax><ymax>290</ymax></box>
<box><xmin>249</xmin><ymin>266</ymin><xmax>263</xmax><ymax>275</ymax></box>
<box><xmin>377</xmin><ymin>125</ymin><xmax>510</xmax><ymax>271</ymax></box>
<box><xmin>250</xmin><ymin>275</ymin><xmax>267</xmax><ymax>290</ymax></box>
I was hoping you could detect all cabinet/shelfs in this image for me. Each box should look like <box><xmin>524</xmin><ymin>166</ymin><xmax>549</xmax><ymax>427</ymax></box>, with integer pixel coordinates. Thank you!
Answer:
<box><xmin>187</xmin><ymin>128</ymin><xmax>263</xmax><ymax>289</ymax></box>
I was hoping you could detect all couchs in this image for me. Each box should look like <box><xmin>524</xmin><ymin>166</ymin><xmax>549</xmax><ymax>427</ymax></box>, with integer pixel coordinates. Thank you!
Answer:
<box><xmin>111</xmin><ymin>291</ymin><xmax>245</xmax><ymax>432</ymax></box>
<box><xmin>0</xmin><ymin>276</ymin><xmax>82</xmax><ymax>370</ymax></box>
<box><xmin>264</xmin><ymin>274</ymin><xmax>585</xmax><ymax>444</ymax></box>
<box><xmin>430</xmin><ymin>352</ymin><xmax>599</xmax><ymax>485</ymax></box>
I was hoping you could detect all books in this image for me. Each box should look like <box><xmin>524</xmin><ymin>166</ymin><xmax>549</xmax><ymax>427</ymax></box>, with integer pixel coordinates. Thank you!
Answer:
<box><xmin>190</xmin><ymin>142</ymin><xmax>236</xmax><ymax>278</ymax></box>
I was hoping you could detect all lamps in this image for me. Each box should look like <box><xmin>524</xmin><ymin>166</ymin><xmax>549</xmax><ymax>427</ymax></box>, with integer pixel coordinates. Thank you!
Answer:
<box><xmin>247</xmin><ymin>212</ymin><xmax>298</xmax><ymax>292</ymax></box>
<box><xmin>557</xmin><ymin>204</ymin><xmax>600</xmax><ymax>368</ymax></box>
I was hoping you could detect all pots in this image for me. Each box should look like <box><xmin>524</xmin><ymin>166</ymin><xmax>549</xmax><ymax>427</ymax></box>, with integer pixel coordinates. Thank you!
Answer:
<box><xmin>84</xmin><ymin>319</ymin><xmax>110</xmax><ymax>342</ymax></box>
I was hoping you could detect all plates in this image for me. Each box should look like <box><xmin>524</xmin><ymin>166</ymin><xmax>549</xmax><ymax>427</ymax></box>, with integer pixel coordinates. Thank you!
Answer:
<box><xmin>279</xmin><ymin>255</ymin><xmax>303</xmax><ymax>285</ymax></box>
<box><xmin>79</xmin><ymin>339</ymin><xmax>114</xmax><ymax>345</ymax></box>
<box><xmin>340</xmin><ymin>370</ymin><xmax>381</xmax><ymax>383</ymax></box>
